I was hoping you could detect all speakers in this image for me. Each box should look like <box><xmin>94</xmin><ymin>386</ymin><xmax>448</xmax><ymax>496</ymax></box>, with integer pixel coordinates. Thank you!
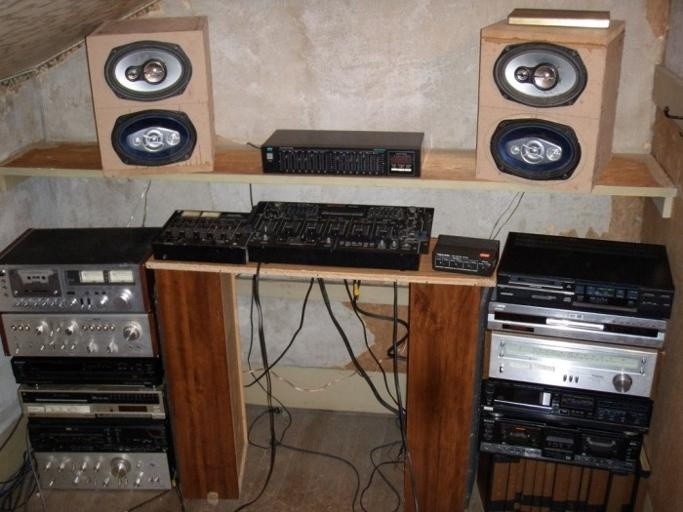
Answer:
<box><xmin>83</xmin><ymin>17</ymin><xmax>216</xmax><ymax>176</ymax></box>
<box><xmin>473</xmin><ymin>19</ymin><xmax>628</xmax><ymax>196</ymax></box>
